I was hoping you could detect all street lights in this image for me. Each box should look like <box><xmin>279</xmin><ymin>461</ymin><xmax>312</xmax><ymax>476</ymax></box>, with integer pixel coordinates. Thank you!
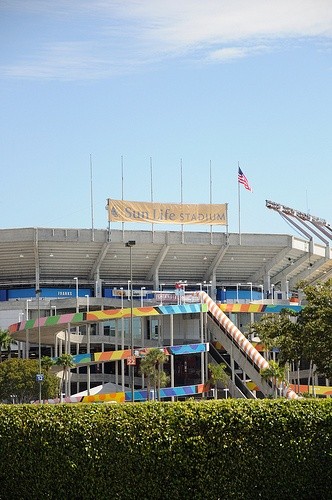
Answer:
<box><xmin>36</xmin><ymin>289</ymin><xmax>43</xmax><ymax>403</ymax></box>
<box><xmin>125</xmin><ymin>240</ymin><xmax>136</xmax><ymax>402</ymax></box>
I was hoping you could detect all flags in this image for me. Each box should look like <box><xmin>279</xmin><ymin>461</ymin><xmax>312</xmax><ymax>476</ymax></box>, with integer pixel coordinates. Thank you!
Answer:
<box><xmin>238</xmin><ymin>167</ymin><xmax>252</xmax><ymax>191</ymax></box>
